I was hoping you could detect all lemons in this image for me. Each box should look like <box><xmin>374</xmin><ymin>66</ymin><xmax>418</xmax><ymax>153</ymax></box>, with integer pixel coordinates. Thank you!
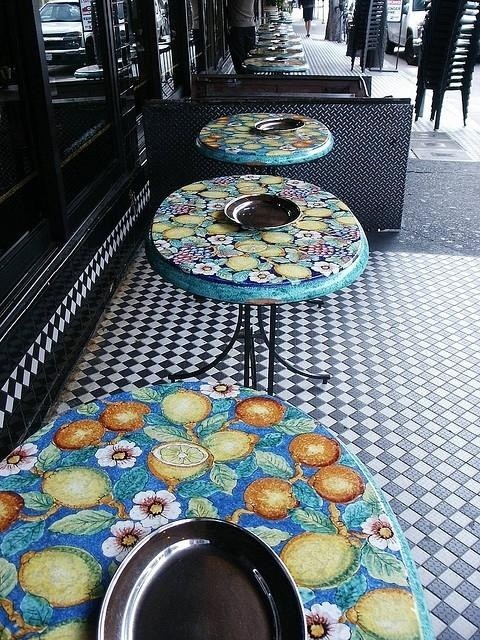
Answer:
<box><xmin>152</xmin><ymin>175</ymin><xmax>356</xmax><ymax>280</ymax></box>
<box><xmin>200</xmin><ymin>113</ymin><xmax>321</xmax><ymax>156</ymax></box>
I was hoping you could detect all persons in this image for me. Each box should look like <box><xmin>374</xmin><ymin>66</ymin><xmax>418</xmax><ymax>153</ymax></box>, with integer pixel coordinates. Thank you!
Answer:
<box><xmin>298</xmin><ymin>0</ymin><xmax>315</xmax><ymax>38</ymax></box>
<box><xmin>227</xmin><ymin>0</ymin><xmax>257</xmax><ymax>78</ymax></box>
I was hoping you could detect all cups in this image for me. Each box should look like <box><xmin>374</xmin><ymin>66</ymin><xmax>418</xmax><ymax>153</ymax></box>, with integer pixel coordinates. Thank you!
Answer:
<box><xmin>256</xmin><ymin>117</ymin><xmax>306</xmax><ymax>135</ymax></box>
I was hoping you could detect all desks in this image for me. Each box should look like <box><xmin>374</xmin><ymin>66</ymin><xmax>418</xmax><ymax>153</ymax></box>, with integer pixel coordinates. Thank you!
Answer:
<box><xmin>197</xmin><ymin>114</ymin><xmax>333</xmax><ymax>177</ymax></box>
<box><xmin>147</xmin><ymin>175</ymin><xmax>369</xmax><ymax>394</ymax></box>
<box><xmin>241</xmin><ymin>11</ymin><xmax>308</xmax><ymax>76</ymax></box>
<box><xmin>0</xmin><ymin>382</ymin><xmax>433</xmax><ymax>640</ymax></box>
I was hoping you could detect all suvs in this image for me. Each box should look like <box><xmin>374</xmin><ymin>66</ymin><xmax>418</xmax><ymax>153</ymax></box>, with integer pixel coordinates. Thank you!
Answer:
<box><xmin>385</xmin><ymin>0</ymin><xmax>432</xmax><ymax>67</ymax></box>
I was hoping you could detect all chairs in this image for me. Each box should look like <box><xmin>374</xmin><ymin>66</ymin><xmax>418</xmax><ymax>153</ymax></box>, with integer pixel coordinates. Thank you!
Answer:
<box><xmin>415</xmin><ymin>0</ymin><xmax>480</xmax><ymax>130</ymax></box>
<box><xmin>346</xmin><ymin>0</ymin><xmax>384</xmax><ymax>71</ymax></box>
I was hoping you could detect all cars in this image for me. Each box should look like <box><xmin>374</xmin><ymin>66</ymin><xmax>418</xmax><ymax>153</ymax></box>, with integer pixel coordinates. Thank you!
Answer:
<box><xmin>39</xmin><ymin>1</ymin><xmax>98</xmax><ymax>66</ymax></box>
<box><xmin>112</xmin><ymin>1</ymin><xmax>166</xmax><ymax>38</ymax></box>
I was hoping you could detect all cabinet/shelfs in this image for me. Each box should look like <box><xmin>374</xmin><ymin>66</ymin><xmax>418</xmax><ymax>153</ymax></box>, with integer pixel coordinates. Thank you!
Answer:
<box><xmin>70</xmin><ymin>34</ymin><xmax>171</xmax><ymax>80</ymax></box>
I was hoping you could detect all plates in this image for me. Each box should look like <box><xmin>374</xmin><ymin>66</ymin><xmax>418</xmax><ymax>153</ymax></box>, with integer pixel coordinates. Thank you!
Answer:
<box><xmin>270</xmin><ymin>39</ymin><xmax>288</xmax><ymax>44</ymax></box>
<box><xmin>264</xmin><ymin>57</ymin><xmax>290</xmax><ymax>63</ymax></box>
<box><xmin>225</xmin><ymin>194</ymin><xmax>302</xmax><ymax>231</ymax></box>
<box><xmin>97</xmin><ymin>517</ymin><xmax>309</xmax><ymax>640</ymax></box>
